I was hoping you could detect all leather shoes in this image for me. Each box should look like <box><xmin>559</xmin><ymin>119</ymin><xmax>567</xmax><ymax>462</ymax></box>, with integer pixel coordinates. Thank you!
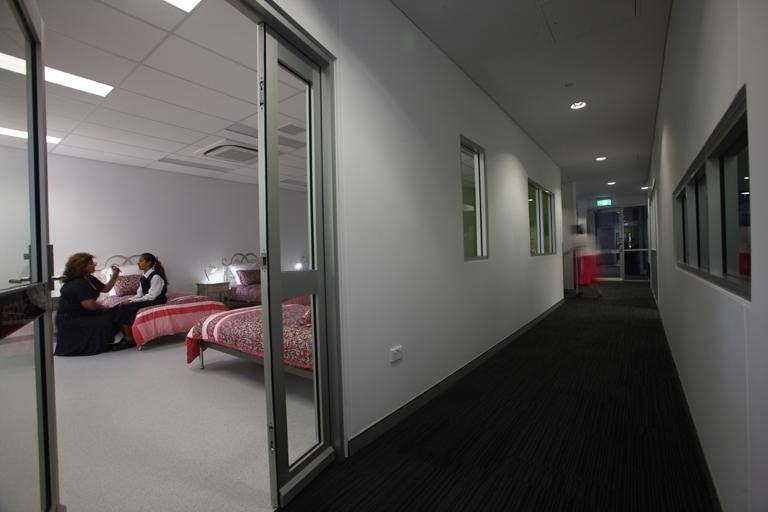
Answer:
<box><xmin>111</xmin><ymin>338</ymin><xmax>135</xmax><ymax>351</ymax></box>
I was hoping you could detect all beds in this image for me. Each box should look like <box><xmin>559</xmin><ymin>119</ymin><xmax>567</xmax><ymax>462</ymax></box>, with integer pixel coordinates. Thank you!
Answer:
<box><xmin>95</xmin><ymin>253</ymin><xmax>313</xmax><ymax>384</ymax></box>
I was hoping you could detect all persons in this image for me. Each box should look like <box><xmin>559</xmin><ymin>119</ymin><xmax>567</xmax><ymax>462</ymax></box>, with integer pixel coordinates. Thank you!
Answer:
<box><xmin>108</xmin><ymin>253</ymin><xmax>168</xmax><ymax>351</ymax></box>
<box><xmin>563</xmin><ymin>225</ymin><xmax>605</xmax><ymax>304</ymax></box>
<box><xmin>53</xmin><ymin>253</ymin><xmax>120</xmax><ymax>357</ymax></box>
